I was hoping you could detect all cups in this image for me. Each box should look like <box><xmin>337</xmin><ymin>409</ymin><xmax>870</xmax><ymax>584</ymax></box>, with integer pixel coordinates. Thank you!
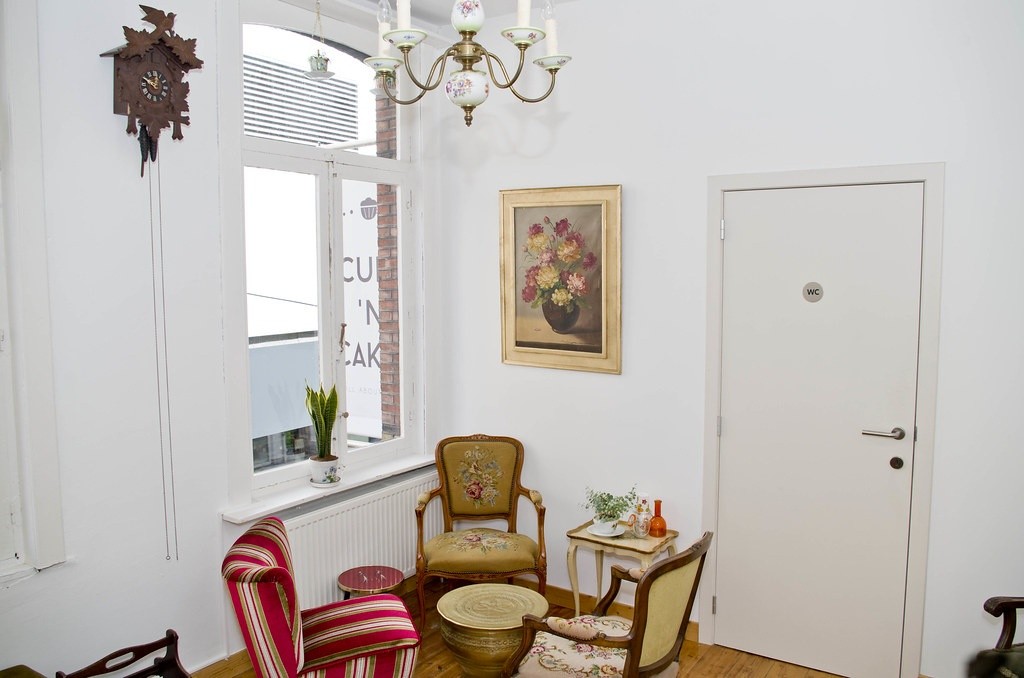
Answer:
<box><xmin>635</xmin><ymin>495</ymin><xmax>648</xmax><ymax>539</ymax></box>
<box><xmin>309</xmin><ymin>57</ymin><xmax>329</xmax><ymax>72</ymax></box>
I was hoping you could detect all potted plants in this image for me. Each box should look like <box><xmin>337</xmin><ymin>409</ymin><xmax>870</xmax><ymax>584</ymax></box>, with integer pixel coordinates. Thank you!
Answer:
<box><xmin>304</xmin><ymin>378</ymin><xmax>342</xmax><ymax>488</ymax></box>
<box><xmin>583</xmin><ymin>485</ymin><xmax>640</xmax><ymax>536</ymax></box>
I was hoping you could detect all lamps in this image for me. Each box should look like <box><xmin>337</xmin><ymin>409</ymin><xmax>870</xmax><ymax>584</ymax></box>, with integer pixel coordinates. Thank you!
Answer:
<box><xmin>363</xmin><ymin>0</ymin><xmax>574</xmax><ymax>128</ymax></box>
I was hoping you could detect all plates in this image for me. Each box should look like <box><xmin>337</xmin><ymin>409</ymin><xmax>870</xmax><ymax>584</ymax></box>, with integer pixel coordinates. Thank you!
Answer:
<box><xmin>303</xmin><ymin>70</ymin><xmax>335</xmax><ymax>80</ymax></box>
<box><xmin>586</xmin><ymin>524</ymin><xmax>626</xmax><ymax>536</ymax></box>
<box><xmin>309</xmin><ymin>477</ymin><xmax>341</xmax><ymax>487</ymax></box>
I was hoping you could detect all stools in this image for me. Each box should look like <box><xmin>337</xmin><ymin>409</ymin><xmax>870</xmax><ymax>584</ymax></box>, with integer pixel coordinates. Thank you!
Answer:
<box><xmin>338</xmin><ymin>566</ymin><xmax>405</xmax><ymax>601</ymax></box>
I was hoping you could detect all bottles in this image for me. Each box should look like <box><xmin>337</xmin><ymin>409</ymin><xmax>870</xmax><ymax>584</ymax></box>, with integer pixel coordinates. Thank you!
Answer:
<box><xmin>649</xmin><ymin>500</ymin><xmax>667</xmax><ymax>537</ymax></box>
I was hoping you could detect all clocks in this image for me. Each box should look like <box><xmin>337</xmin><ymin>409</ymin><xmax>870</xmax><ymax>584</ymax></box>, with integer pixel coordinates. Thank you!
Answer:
<box><xmin>99</xmin><ymin>4</ymin><xmax>205</xmax><ymax>179</ymax></box>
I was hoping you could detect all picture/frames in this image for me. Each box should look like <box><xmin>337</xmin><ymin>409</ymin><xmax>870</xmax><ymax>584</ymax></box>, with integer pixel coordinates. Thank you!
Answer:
<box><xmin>499</xmin><ymin>184</ymin><xmax>623</xmax><ymax>375</ymax></box>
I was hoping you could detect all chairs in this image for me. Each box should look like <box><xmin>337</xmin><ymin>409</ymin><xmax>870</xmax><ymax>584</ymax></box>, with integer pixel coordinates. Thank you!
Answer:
<box><xmin>414</xmin><ymin>434</ymin><xmax>548</xmax><ymax>648</ymax></box>
<box><xmin>221</xmin><ymin>516</ymin><xmax>420</xmax><ymax>678</ymax></box>
<box><xmin>500</xmin><ymin>531</ymin><xmax>714</xmax><ymax>678</ymax></box>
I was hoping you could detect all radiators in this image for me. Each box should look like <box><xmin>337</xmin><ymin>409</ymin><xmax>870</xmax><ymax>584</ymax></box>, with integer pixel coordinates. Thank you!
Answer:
<box><xmin>282</xmin><ymin>470</ymin><xmax>458</xmax><ymax>612</ymax></box>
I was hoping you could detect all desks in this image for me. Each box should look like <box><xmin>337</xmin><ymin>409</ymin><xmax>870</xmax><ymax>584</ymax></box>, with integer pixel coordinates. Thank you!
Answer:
<box><xmin>436</xmin><ymin>583</ymin><xmax>550</xmax><ymax>678</ymax></box>
<box><xmin>567</xmin><ymin>518</ymin><xmax>679</xmax><ymax>618</ymax></box>
